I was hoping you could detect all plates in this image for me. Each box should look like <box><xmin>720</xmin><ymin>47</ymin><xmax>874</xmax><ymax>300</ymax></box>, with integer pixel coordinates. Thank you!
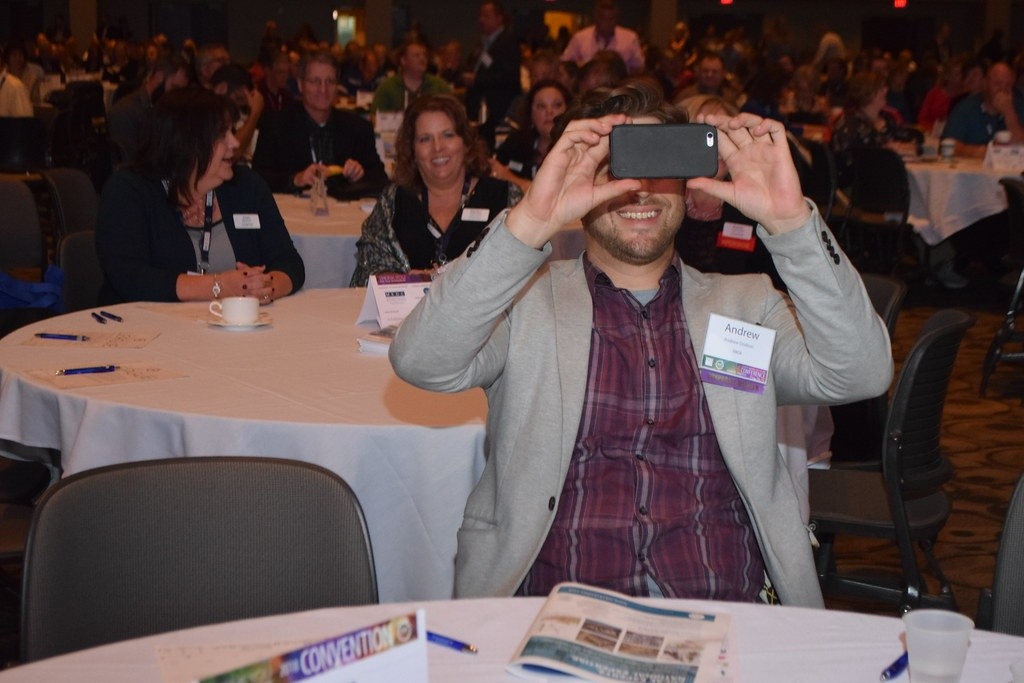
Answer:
<box><xmin>207</xmin><ymin>316</ymin><xmax>271</xmax><ymax>331</ymax></box>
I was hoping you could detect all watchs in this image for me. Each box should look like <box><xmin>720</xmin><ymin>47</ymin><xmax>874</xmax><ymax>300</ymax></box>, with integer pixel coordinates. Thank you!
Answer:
<box><xmin>213</xmin><ymin>273</ymin><xmax>221</xmax><ymax>299</ymax></box>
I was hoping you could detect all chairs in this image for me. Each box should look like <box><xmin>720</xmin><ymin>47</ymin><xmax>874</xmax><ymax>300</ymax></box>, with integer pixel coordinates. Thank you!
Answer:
<box><xmin>1</xmin><ymin>118</ymin><xmax>1024</xmax><ymax>661</ymax></box>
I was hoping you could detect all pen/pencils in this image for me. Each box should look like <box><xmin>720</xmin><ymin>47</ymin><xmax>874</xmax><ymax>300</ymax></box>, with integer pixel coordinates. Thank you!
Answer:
<box><xmin>100</xmin><ymin>309</ymin><xmax>123</xmax><ymax>323</ymax></box>
<box><xmin>310</xmin><ymin>144</ymin><xmax>318</xmax><ymax>175</ymax></box>
<box><xmin>91</xmin><ymin>312</ymin><xmax>106</xmax><ymax>324</ymax></box>
<box><xmin>35</xmin><ymin>332</ymin><xmax>90</xmax><ymax>342</ymax></box>
<box><xmin>881</xmin><ymin>649</ymin><xmax>908</xmax><ymax>681</ymax></box>
<box><xmin>425</xmin><ymin>630</ymin><xmax>478</xmax><ymax>657</ymax></box>
<box><xmin>55</xmin><ymin>364</ymin><xmax>121</xmax><ymax>376</ymax></box>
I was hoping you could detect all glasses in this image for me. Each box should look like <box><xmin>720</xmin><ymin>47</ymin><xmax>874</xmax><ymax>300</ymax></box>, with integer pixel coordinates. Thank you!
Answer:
<box><xmin>302</xmin><ymin>74</ymin><xmax>337</xmax><ymax>86</ymax></box>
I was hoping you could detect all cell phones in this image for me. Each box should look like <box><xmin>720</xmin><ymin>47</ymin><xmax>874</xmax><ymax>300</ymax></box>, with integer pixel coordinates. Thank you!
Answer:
<box><xmin>609</xmin><ymin>123</ymin><xmax>718</xmax><ymax>178</ymax></box>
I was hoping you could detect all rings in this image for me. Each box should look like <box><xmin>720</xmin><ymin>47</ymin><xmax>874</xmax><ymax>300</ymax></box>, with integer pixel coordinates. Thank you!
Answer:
<box><xmin>265</xmin><ymin>295</ymin><xmax>269</xmax><ymax>299</ymax></box>
<box><xmin>493</xmin><ymin>172</ymin><xmax>497</xmax><ymax>176</ymax></box>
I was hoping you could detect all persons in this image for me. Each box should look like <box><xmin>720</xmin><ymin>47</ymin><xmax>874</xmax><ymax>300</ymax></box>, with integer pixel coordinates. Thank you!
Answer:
<box><xmin>350</xmin><ymin>92</ymin><xmax>525</xmax><ymax>287</ymax></box>
<box><xmin>388</xmin><ymin>85</ymin><xmax>893</xmax><ymax>609</ymax></box>
<box><xmin>95</xmin><ymin>93</ymin><xmax>306</xmax><ymax>305</ymax></box>
<box><xmin>1</xmin><ymin>0</ymin><xmax>1024</xmax><ymax>286</ymax></box>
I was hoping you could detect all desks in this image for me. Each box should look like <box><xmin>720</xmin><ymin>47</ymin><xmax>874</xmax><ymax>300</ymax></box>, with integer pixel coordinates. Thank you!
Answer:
<box><xmin>270</xmin><ymin>190</ymin><xmax>377</xmax><ymax>289</ymax></box>
<box><xmin>896</xmin><ymin>153</ymin><xmax>1024</xmax><ymax>273</ymax></box>
<box><xmin>0</xmin><ymin>596</ymin><xmax>1024</xmax><ymax>683</ymax></box>
<box><xmin>0</xmin><ymin>293</ymin><xmax>491</xmax><ymax>605</ymax></box>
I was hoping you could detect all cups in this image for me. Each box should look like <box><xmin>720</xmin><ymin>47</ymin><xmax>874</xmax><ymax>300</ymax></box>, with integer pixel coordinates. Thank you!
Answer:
<box><xmin>209</xmin><ymin>296</ymin><xmax>259</xmax><ymax>324</ymax></box>
<box><xmin>901</xmin><ymin>609</ymin><xmax>975</xmax><ymax>683</ymax></box>
<box><xmin>940</xmin><ymin>139</ymin><xmax>955</xmax><ymax>158</ymax></box>
